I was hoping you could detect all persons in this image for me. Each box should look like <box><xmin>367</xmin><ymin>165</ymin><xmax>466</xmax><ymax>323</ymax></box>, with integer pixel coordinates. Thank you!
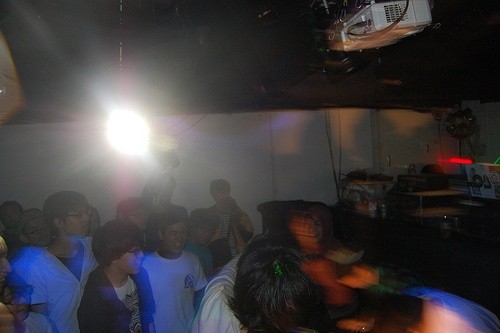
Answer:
<box><xmin>1</xmin><ymin>177</ymin><xmax>500</xmax><ymax>332</ymax></box>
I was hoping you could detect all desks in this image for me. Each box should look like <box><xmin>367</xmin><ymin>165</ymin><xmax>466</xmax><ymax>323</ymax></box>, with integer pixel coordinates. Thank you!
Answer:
<box><xmin>343</xmin><ymin>178</ymin><xmax>396</xmax><ymax>204</ymax></box>
<box><xmin>466</xmin><ymin>162</ymin><xmax>500</xmax><ymax>200</ymax></box>
<box><xmin>403</xmin><ymin>190</ymin><xmax>471</xmax><ymax>210</ymax></box>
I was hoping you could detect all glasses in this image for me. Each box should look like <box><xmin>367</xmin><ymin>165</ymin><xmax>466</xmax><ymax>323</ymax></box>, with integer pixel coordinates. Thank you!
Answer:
<box><xmin>127</xmin><ymin>249</ymin><xmax>140</xmax><ymax>255</ymax></box>
<box><xmin>27</xmin><ymin>229</ymin><xmax>39</xmax><ymax>235</ymax></box>
<box><xmin>68</xmin><ymin>208</ymin><xmax>93</xmax><ymax>218</ymax></box>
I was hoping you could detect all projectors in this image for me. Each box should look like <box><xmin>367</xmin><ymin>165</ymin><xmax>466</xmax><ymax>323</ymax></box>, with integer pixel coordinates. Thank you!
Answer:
<box><xmin>324</xmin><ymin>2</ymin><xmax>432</xmax><ymax>51</ymax></box>
